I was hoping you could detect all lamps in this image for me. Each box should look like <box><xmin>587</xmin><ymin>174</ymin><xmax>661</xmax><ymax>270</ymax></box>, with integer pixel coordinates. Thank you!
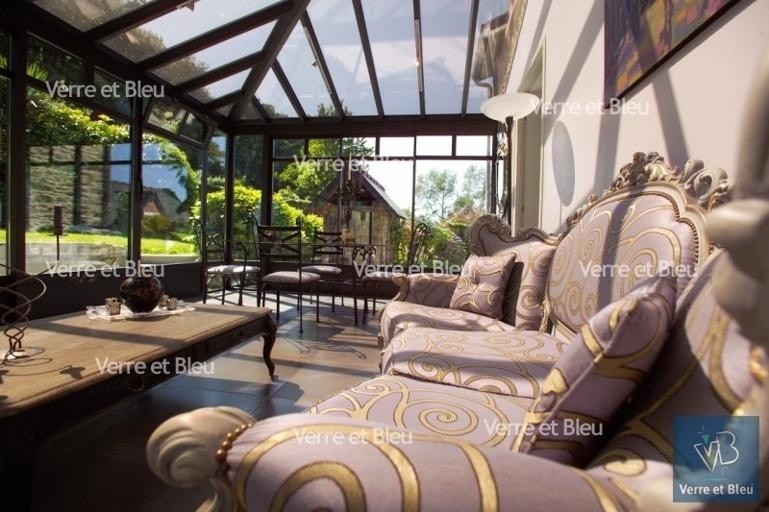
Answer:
<box><xmin>480</xmin><ymin>92</ymin><xmax>541</xmax><ymax>238</ymax></box>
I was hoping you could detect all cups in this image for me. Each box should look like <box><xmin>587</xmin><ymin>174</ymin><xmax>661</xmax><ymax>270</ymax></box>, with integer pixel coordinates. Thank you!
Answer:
<box><xmin>105</xmin><ymin>297</ymin><xmax>122</xmax><ymax>315</ymax></box>
<box><xmin>160</xmin><ymin>295</ymin><xmax>177</xmax><ymax>310</ymax></box>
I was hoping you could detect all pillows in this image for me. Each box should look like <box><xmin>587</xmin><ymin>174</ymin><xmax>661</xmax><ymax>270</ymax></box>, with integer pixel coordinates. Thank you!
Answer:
<box><xmin>510</xmin><ymin>265</ymin><xmax>678</xmax><ymax>470</ymax></box>
<box><xmin>449</xmin><ymin>252</ymin><xmax>517</xmax><ymax>320</ymax></box>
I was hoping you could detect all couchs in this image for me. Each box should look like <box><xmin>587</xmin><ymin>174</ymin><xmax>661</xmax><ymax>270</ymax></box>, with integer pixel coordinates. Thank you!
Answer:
<box><xmin>145</xmin><ymin>151</ymin><xmax>769</xmax><ymax>512</ymax></box>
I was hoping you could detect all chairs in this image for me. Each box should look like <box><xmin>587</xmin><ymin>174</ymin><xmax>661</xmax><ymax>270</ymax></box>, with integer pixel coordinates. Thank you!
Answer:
<box><xmin>189</xmin><ymin>213</ymin><xmax>429</xmax><ymax>333</ymax></box>
